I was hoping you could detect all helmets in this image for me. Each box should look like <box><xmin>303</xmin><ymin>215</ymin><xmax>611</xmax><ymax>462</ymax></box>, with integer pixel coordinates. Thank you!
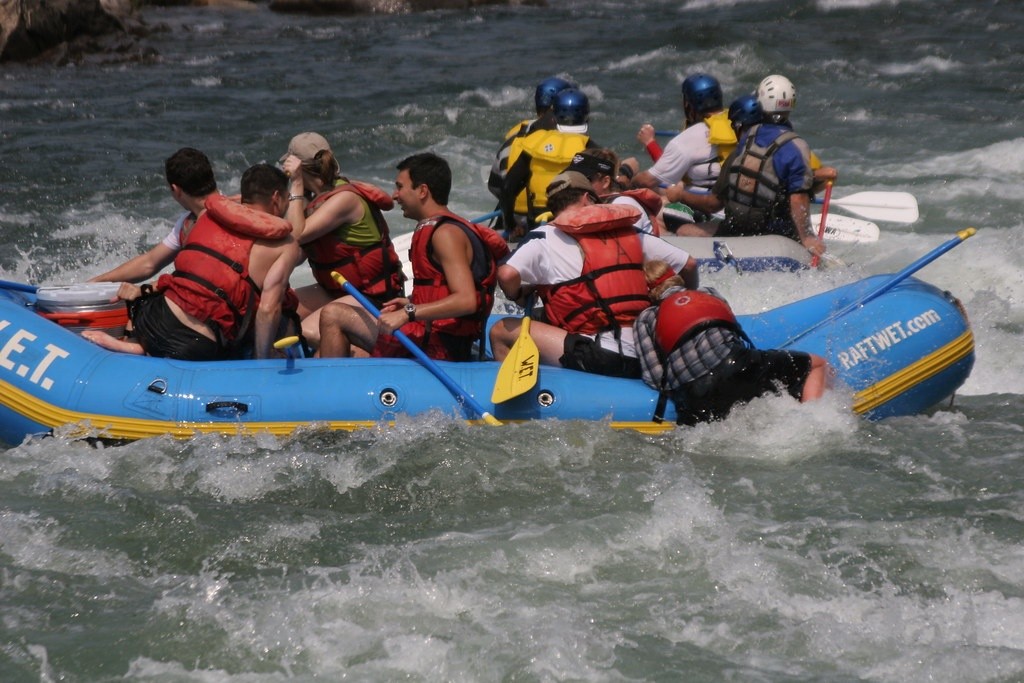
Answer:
<box><xmin>728</xmin><ymin>97</ymin><xmax>762</xmax><ymax>140</ymax></box>
<box><xmin>553</xmin><ymin>88</ymin><xmax>589</xmax><ymax>124</ymax></box>
<box><xmin>757</xmin><ymin>75</ymin><xmax>796</xmax><ymax>110</ymax></box>
<box><xmin>682</xmin><ymin>73</ymin><xmax>720</xmax><ymax>122</ymax></box>
<box><xmin>535</xmin><ymin>78</ymin><xmax>572</xmax><ymax>115</ymax></box>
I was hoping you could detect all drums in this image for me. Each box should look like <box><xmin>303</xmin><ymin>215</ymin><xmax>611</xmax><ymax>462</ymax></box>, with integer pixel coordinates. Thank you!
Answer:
<box><xmin>36</xmin><ymin>281</ymin><xmax>129</xmax><ymax>343</ymax></box>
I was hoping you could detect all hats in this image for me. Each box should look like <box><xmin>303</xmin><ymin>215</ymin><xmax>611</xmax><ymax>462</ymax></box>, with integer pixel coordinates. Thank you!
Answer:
<box><xmin>557</xmin><ymin>153</ymin><xmax>614</xmax><ymax>179</ymax></box>
<box><xmin>545</xmin><ymin>171</ymin><xmax>604</xmax><ymax>204</ymax></box>
<box><xmin>279</xmin><ymin>132</ymin><xmax>330</xmax><ymax>163</ymax></box>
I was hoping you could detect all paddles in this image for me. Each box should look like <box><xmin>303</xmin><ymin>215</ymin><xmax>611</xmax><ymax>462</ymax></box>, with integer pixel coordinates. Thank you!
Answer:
<box><xmin>489</xmin><ymin>288</ymin><xmax>537</xmax><ymax>404</ymax></box>
<box><xmin>808</xmin><ymin>212</ymin><xmax>882</xmax><ymax>245</ymax></box>
<box><xmin>654</xmin><ymin>182</ymin><xmax>920</xmax><ymax>226</ymax></box>
<box><xmin>775</xmin><ymin>224</ymin><xmax>978</xmax><ymax>351</ymax></box>
<box><xmin>812</xmin><ymin>174</ymin><xmax>838</xmax><ymax>266</ymax></box>
<box><xmin>336</xmin><ymin>266</ymin><xmax>506</xmax><ymax>427</ymax></box>
<box><xmin>461</xmin><ymin>211</ymin><xmax>507</xmax><ymax>225</ymax></box>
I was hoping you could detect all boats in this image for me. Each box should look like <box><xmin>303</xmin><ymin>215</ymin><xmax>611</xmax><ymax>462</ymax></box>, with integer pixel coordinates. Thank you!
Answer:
<box><xmin>2</xmin><ymin>226</ymin><xmax>978</xmax><ymax>449</ymax></box>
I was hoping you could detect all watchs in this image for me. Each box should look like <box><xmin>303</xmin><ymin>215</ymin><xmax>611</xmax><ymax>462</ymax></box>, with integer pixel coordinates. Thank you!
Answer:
<box><xmin>288</xmin><ymin>193</ymin><xmax>304</xmax><ymax>201</ymax></box>
<box><xmin>404</xmin><ymin>303</ymin><xmax>415</xmax><ymax>322</ymax></box>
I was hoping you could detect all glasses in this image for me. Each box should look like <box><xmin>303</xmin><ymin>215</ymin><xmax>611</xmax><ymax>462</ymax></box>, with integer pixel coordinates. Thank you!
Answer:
<box><xmin>287</xmin><ymin>194</ymin><xmax>292</xmax><ymax>200</ymax></box>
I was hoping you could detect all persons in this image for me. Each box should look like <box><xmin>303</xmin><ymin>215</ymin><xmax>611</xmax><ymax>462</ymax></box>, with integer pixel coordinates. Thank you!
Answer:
<box><xmin>88</xmin><ymin>132</ymin><xmax>497</xmax><ymax>362</ymax></box>
<box><xmin>620</xmin><ymin>73</ymin><xmax>836</xmax><ymax>258</ymax></box>
<box><xmin>488</xmin><ymin>78</ymin><xmax>699</xmax><ymax>379</ymax></box>
<box><xmin>632</xmin><ymin>261</ymin><xmax>829</xmax><ymax>427</ymax></box>
